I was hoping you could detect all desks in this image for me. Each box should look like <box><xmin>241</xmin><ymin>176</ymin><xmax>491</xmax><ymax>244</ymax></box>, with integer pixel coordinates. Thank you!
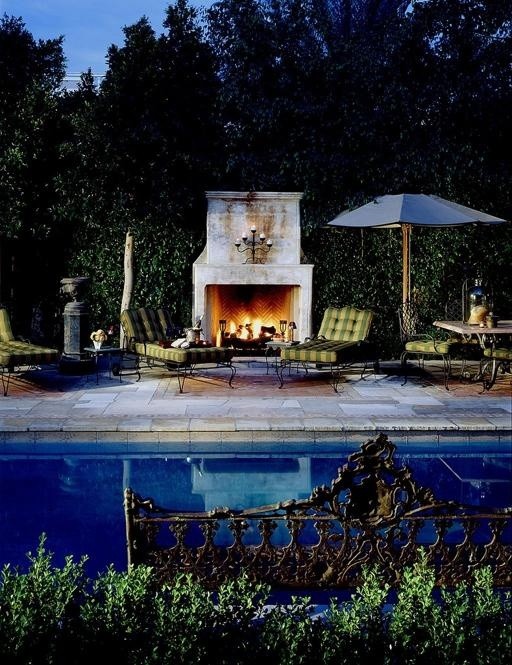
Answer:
<box><xmin>433</xmin><ymin>320</ymin><xmax>512</xmax><ymax>395</ymax></box>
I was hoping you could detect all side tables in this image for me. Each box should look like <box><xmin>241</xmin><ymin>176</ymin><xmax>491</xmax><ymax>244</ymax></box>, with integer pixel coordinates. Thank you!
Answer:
<box><xmin>264</xmin><ymin>341</ymin><xmax>301</xmax><ymax>376</ymax></box>
<box><xmin>84</xmin><ymin>346</ymin><xmax>123</xmax><ymax>385</ymax></box>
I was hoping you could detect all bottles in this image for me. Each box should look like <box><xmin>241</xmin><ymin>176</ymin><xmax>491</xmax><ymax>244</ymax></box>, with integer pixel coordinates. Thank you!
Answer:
<box><xmin>194</xmin><ymin>314</ymin><xmax>204</xmax><ymax>328</ymax></box>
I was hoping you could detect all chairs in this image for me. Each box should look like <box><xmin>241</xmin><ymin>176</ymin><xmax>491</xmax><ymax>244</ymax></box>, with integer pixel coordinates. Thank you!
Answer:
<box><xmin>0</xmin><ymin>307</ymin><xmax>60</xmax><ymax>398</ymax></box>
<box><xmin>485</xmin><ymin>347</ymin><xmax>512</xmax><ymax>390</ymax></box>
<box><xmin>398</xmin><ymin>301</ymin><xmax>453</xmax><ymax>391</ymax></box>
<box><xmin>277</xmin><ymin>305</ymin><xmax>374</xmax><ymax>394</ymax></box>
<box><xmin>121</xmin><ymin>308</ymin><xmax>235</xmax><ymax>394</ymax></box>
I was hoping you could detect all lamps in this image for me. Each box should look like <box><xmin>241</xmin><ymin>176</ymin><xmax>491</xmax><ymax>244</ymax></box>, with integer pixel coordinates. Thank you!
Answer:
<box><xmin>287</xmin><ymin>322</ymin><xmax>298</xmax><ymax>344</ymax></box>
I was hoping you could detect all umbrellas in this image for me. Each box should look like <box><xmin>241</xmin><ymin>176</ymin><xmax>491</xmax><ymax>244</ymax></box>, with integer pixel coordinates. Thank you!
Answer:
<box><xmin>327</xmin><ymin>194</ymin><xmax>507</xmax><ymax>345</ymax></box>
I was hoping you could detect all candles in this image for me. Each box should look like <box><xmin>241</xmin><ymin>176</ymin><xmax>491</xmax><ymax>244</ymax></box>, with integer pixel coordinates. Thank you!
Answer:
<box><xmin>267</xmin><ymin>240</ymin><xmax>272</xmax><ymax>244</ymax></box>
<box><xmin>242</xmin><ymin>232</ymin><xmax>248</xmax><ymax>238</ymax></box>
<box><xmin>260</xmin><ymin>234</ymin><xmax>265</xmax><ymax>238</ymax></box>
<box><xmin>235</xmin><ymin>240</ymin><xmax>240</xmax><ymax>244</ymax></box>
<box><xmin>251</xmin><ymin>226</ymin><xmax>256</xmax><ymax>230</ymax></box>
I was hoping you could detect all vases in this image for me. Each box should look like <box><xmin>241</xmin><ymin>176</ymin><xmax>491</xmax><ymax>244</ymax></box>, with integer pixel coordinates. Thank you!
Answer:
<box><xmin>93</xmin><ymin>341</ymin><xmax>101</xmax><ymax>348</ymax></box>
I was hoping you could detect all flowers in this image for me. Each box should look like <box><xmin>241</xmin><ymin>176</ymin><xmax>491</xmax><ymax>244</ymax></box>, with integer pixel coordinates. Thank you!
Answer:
<box><xmin>90</xmin><ymin>329</ymin><xmax>106</xmax><ymax>341</ymax></box>
